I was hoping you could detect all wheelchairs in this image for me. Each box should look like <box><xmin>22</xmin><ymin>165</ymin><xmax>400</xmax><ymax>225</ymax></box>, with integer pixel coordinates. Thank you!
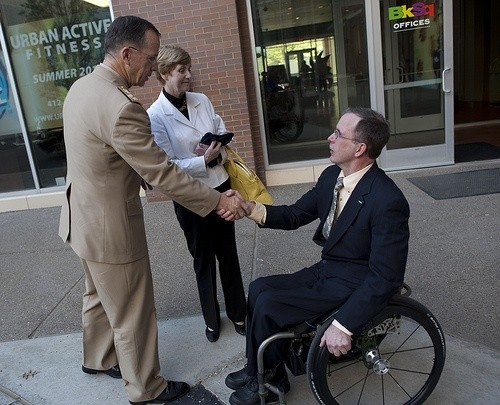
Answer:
<box><xmin>257</xmin><ymin>281</ymin><xmax>446</xmax><ymax>405</ymax></box>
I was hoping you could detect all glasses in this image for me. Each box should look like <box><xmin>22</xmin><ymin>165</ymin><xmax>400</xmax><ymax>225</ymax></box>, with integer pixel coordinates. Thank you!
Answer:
<box><xmin>129</xmin><ymin>47</ymin><xmax>159</xmax><ymax>66</ymax></box>
<box><xmin>334</xmin><ymin>130</ymin><xmax>368</xmax><ymax>153</ymax></box>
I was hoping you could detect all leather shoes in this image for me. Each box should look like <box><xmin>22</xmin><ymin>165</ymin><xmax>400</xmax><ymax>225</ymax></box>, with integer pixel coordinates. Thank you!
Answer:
<box><xmin>82</xmin><ymin>364</ymin><xmax>122</xmax><ymax>379</ymax></box>
<box><xmin>129</xmin><ymin>380</ymin><xmax>190</xmax><ymax>405</ymax></box>
<box><xmin>225</xmin><ymin>364</ymin><xmax>269</xmax><ymax>390</ymax></box>
<box><xmin>206</xmin><ymin>326</ymin><xmax>219</xmax><ymax>342</ymax></box>
<box><xmin>229</xmin><ymin>362</ymin><xmax>290</xmax><ymax>405</ymax></box>
<box><xmin>232</xmin><ymin>320</ymin><xmax>246</xmax><ymax>335</ymax></box>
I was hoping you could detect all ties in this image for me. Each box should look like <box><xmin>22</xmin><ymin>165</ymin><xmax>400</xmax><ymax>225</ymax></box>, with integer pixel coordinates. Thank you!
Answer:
<box><xmin>322</xmin><ymin>177</ymin><xmax>344</xmax><ymax>241</ymax></box>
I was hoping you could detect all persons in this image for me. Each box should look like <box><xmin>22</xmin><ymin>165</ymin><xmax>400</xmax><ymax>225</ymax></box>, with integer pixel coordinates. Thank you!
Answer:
<box><xmin>56</xmin><ymin>15</ymin><xmax>249</xmax><ymax>405</ymax></box>
<box><xmin>217</xmin><ymin>108</ymin><xmax>410</xmax><ymax>405</ymax></box>
<box><xmin>144</xmin><ymin>46</ymin><xmax>246</xmax><ymax>343</ymax></box>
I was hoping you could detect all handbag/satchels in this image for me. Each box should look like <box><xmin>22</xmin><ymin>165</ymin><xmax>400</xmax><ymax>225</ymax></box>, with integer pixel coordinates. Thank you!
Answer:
<box><xmin>224</xmin><ymin>145</ymin><xmax>273</xmax><ymax>207</ymax></box>
